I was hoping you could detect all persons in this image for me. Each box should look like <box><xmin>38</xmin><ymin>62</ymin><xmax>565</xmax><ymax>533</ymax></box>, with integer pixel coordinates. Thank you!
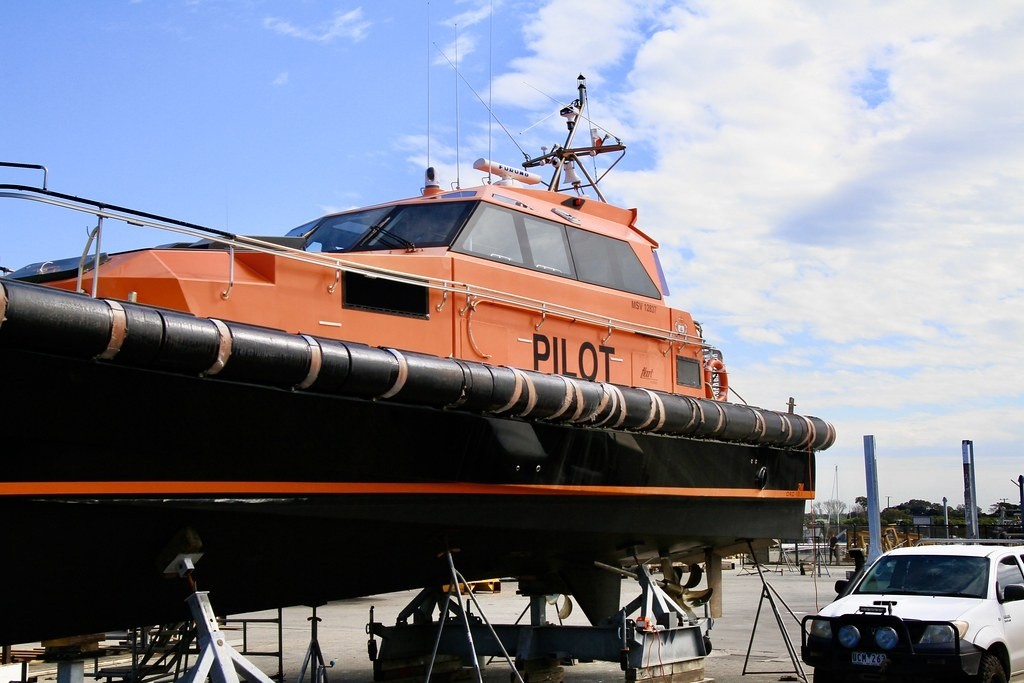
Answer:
<box><xmin>826</xmin><ymin>533</ymin><xmax>839</xmax><ymax>565</ymax></box>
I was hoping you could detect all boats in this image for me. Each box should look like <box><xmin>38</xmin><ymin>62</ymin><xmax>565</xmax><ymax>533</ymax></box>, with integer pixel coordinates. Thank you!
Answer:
<box><xmin>1</xmin><ymin>71</ymin><xmax>836</xmax><ymax>646</ymax></box>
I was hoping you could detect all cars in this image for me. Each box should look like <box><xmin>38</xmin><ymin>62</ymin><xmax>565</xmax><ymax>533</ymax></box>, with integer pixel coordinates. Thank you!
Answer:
<box><xmin>801</xmin><ymin>538</ymin><xmax>1024</xmax><ymax>683</ymax></box>
